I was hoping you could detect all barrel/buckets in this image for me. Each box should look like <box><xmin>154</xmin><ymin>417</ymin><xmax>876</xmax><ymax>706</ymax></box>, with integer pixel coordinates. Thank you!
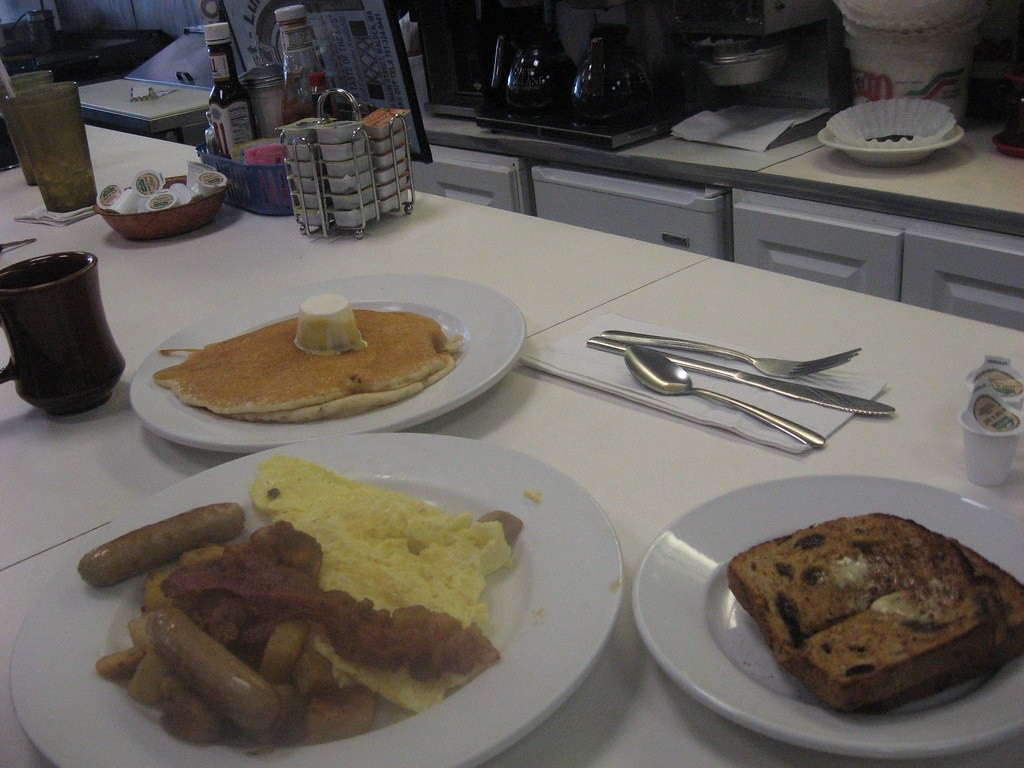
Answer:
<box><xmin>841</xmin><ymin>14</ymin><xmax>981</xmax><ymax>125</ymax></box>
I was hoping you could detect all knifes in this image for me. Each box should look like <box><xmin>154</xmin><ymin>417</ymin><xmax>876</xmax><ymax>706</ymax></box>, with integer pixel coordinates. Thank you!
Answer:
<box><xmin>586</xmin><ymin>335</ymin><xmax>896</xmax><ymax>417</ymax></box>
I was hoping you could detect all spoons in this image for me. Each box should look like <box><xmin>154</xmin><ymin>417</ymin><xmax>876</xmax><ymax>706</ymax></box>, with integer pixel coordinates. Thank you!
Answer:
<box><xmin>622</xmin><ymin>344</ymin><xmax>826</xmax><ymax>448</ymax></box>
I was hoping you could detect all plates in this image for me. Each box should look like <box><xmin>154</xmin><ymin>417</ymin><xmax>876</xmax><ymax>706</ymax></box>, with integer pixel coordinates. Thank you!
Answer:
<box><xmin>129</xmin><ymin>272</ymin><xmax>526</xmax><ymax>453</ymax></box>
<box><xmin>630</xmin><ymin>474</ymin><xmax>1024</xmax><ymax>759</ymax></box>
<box><xmin>11</xmin><ymin>429</ymin><xmax>623</xmax><ymax>768</ymax></box>
<box><xmin>991</xmin><ymin>131</ymin><xmax>1024</xmax><ymax>158</ymax></box>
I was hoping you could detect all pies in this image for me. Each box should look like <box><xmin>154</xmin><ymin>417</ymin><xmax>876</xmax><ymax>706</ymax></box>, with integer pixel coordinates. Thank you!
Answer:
<box><xmin>153</xmin><ymin>310</ymin><xmax>456</xmax><ymax>423</ymax></box>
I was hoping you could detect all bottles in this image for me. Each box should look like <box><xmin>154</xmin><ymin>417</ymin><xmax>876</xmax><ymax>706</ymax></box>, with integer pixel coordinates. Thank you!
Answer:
<box><xmin>203</xmin><ymin>4</ymin><xmax>340</xmax><ymax>158</ymax></box>
<box><xmin>26</xmin><ymin>8</ymin><xmax>58</xmax><ymax>54</ymax></box>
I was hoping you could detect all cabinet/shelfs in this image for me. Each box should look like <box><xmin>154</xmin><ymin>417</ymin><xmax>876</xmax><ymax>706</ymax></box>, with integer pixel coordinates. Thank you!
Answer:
<box><xmin>408</xmin><ymin>146</ymin><xmax>1024</xmax><ymax>330</ymax></box>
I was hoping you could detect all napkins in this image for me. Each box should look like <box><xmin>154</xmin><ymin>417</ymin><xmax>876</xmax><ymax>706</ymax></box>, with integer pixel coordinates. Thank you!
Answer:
<box><xmin>520</xmin><ymin>315</ymin><xmax>888</xmax><ymax>456</ymax></box>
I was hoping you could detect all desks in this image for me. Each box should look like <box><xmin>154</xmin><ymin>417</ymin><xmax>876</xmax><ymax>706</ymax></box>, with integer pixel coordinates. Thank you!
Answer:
<box><xmin>1</xmin><ymin>125</ymin><xmax>1024</xmax><ymax>768</ymax></box>
<box><xmin>75</xmin><ymin>79</ymin><xmax>214</xmax><ymax>142</ymax></box>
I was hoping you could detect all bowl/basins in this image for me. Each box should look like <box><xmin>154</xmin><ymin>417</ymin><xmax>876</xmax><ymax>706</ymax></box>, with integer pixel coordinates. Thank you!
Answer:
<box><xmin>816</xmin><ymin>125</ymin><xmax>965</xmax><ymax>167</ymax></box>
<box><xmin>93</xmin><ymin>175</ymin><xmax>229</xmax><ymax>240</ymax></box>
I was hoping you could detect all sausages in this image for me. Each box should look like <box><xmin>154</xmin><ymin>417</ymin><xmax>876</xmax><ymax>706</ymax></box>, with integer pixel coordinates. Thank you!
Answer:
<box><xmin>79</xmin><ymin>502</ymin><xmax>246</xmax><ymax>586</ymax></box>
<box><xmin>146</xmin><ymin>606</ymin><xmax>279</xmax><ymax>731</ymax></box>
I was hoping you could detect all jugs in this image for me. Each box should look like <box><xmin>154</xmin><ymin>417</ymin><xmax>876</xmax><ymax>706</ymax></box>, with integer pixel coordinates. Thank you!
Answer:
<box><xmin>490</xmin><ymin>22</ymin><xmax>653</xmax><ymax>128</ymax></box>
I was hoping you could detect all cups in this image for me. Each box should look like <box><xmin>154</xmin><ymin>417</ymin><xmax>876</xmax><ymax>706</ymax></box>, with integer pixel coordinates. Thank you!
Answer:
<box><xmin>0</xmin><ymin>70</ymin><xmax>55</xmax><ymax>187</ymax></box>
<box><xmin>0</xmin><ymin>250</ymin><xmax>127</xmax><ymax>420</ymax></box>
<box><xmin>5</xmin><ymin>81</ymin><xmax>97</xmax><ymax>215</ymax></box>
<box><xmin>1000</xmin><ymin>61</ymin><xmax>1024</xmax><ymax>149</ymax></box>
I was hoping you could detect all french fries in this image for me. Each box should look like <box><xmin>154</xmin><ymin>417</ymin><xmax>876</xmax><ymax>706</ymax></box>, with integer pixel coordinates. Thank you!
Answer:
<box><xmin>98</xmin><ymin>543</ymin><xmax>373</xmax><ymax>739</ymax></box>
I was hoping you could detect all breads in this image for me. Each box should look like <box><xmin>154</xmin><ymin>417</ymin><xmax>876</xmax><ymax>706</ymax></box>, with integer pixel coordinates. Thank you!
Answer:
<box><xmin>728</xmin><ymin>512</ymin><xmax>1023</xmax><ymax>715</ymax></box>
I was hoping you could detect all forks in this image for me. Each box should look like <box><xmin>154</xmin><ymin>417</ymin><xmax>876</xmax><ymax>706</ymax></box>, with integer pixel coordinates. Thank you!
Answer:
<box><xmin>601</xmin><ymin>328</ymin><xmax>862</xmax><ymax>381</ymax></box>
